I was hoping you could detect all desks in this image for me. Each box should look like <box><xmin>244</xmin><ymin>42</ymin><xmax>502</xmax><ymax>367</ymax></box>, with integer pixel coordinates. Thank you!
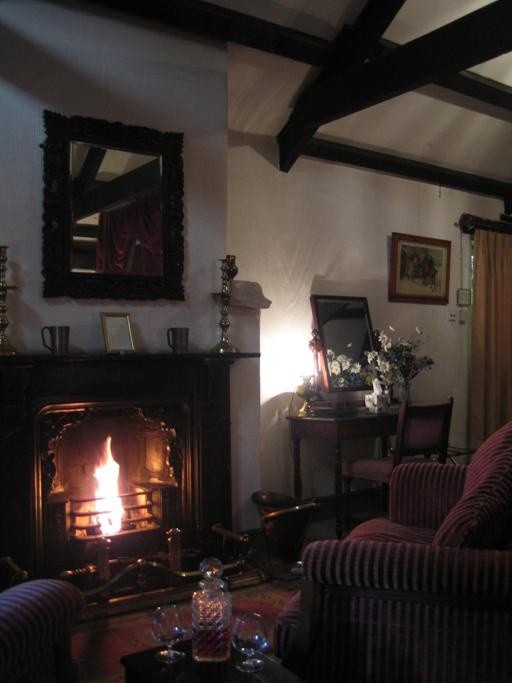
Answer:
<box><xmin>289</xmin><ymin>412</ymin><xmax>399</xmax><ymax>539</ymax></box>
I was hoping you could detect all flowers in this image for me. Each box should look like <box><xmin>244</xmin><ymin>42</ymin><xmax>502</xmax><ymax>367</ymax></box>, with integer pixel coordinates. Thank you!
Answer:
<box><xmin>367</xmin><ymin>321</ymin><xmax>424</xmax><ymax>385</ymax></box>
<box><xmin>323</xmin><ymin>347</ymin><xmax>375</xmax><ymax>389</ymax></box>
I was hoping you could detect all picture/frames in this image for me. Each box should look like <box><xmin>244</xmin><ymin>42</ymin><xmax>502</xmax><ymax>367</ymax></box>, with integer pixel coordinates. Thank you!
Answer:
<box><xmin>388</xmin><ymin>233</ymin><xmax>451</xmax><ymax>305</ymax></box>
<box><xmin>101</xmin><ymin>309</ymin><xmax>136</xmax><ymax>353</ymax></box>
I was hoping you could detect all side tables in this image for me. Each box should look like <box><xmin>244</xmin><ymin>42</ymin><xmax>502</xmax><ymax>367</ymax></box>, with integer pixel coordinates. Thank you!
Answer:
<box><xmin>118</xmin><ymin>633</ymin><xmax>300</xmax><ymax>683</ymax></box>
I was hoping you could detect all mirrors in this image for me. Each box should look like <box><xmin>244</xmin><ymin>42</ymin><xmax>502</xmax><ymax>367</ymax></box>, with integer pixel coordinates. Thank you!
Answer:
<box><xmin>43</xmin><ymin>109</ymin><xmax>188</xmax><ymax>301</ymax></box>
<box><xmin>310</xmin><ymin>294</ymin><xmax>377</xmax><ymax>394</ymax></box>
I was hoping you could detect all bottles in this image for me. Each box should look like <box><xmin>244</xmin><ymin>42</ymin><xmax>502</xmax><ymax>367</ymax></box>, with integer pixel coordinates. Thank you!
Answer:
<box><xmin>192</xmin><ymin>559</ymin><xmax>233</xmax><ymax>661</ymax></box>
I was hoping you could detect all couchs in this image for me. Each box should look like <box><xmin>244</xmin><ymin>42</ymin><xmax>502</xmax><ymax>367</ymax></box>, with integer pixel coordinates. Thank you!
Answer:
<box><xmin>273</xmin><ymin>418</ymin><xmax>512</xmax><ymax>683</ymax></box>
<box><xmin>0</xmin><ymin>575</ymin><xmax>85</xmax><ymax>683</ymax></box>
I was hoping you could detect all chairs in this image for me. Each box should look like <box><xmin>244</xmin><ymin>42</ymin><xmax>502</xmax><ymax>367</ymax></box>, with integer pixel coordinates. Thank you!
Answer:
<box><xmin>342</xmin><ymin>394</ymin><xmax>455</xmax><ymax>531</ymax></box>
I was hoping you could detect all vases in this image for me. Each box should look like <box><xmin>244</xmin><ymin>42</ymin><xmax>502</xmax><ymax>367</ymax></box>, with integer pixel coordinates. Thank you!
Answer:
<box><xmin>393</xmin><ymin>382</ymin><xmax>407</xmax><ymax>405</ymax></box>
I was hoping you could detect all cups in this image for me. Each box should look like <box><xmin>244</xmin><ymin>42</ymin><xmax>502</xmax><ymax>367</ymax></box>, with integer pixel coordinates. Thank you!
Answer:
<box><xmin>41</xmin><ymin>326</ymin><xmax>70</xmax><ymax>354</ymax></box>
<box><xmin>229</xmin><ymin>611</ymin><xmax>271</xmax><ymax>674</ymax></box>
<box><xmin>166</xmin><ymin>327</ymin><xmax>189</xmax><ymax>354</ymax></box>
<box><xmin>151</xmin><ymin>603</ymin><xmax>185</xmax><ymax>664</ymax></box>
